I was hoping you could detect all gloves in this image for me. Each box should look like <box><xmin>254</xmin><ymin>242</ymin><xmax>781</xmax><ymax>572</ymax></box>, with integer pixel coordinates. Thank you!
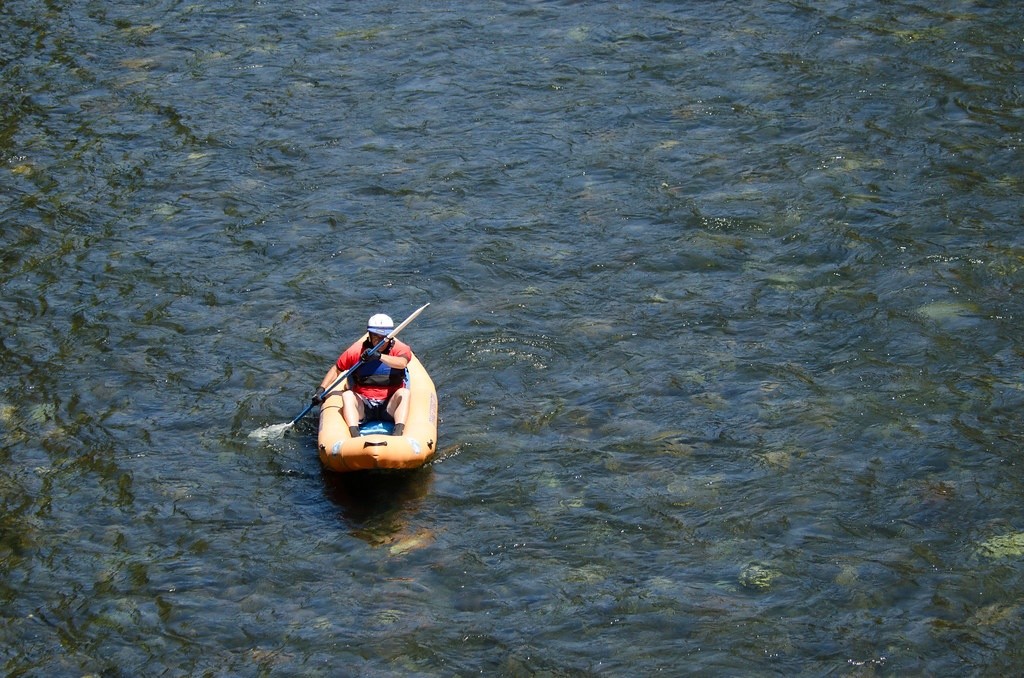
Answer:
<box><xmin>311</xmin><ymin>386</ymin><xmax>325</xmax><ymax>405</ymax></box>
<box><xmin>361</xmin><ymin>350</ymin><xmax>381</xmax><ymax>364</ymax></box>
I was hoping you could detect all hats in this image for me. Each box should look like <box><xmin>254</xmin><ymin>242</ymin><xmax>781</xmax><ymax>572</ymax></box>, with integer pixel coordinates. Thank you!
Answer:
<box><xmin>367</xmin><ymin>314</ymin><xmax>394</xmax><ymax>335</ymax></box>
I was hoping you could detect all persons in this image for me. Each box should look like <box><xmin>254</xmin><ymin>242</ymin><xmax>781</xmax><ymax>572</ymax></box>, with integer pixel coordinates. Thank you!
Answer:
<box><xmin>312</xmin><ymin>314</ymin><xmax>411</xmax><ymax>436</ymax></box>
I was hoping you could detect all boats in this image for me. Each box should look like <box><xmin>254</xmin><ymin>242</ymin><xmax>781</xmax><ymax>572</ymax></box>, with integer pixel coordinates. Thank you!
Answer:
<box><xmin>317</xmin><ymin>351</ymin><xmax>438</xmax><ymax>473</ymax></box>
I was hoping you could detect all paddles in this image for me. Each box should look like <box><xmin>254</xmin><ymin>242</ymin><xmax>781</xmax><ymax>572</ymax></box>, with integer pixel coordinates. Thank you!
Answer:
<box><xmin>287</xmin><ymin>301</ymin><xmax>432</xmax><ymax>428</ymax></box>
